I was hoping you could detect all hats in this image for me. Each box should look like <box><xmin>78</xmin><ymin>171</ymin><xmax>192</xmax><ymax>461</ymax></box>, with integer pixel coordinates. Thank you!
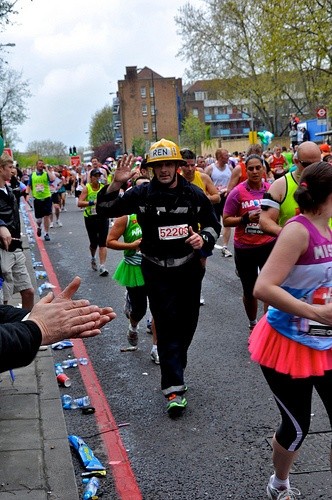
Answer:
<box><xmin>89</xmin><ymin>169</ymin><xmax>103</xmax><ymax>177</ymax></box>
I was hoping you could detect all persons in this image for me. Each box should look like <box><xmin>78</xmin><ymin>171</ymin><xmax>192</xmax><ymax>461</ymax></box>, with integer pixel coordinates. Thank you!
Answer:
<box><xmin>0</xmin><ymin>113</ymin><xmax>332</xmax><ymax>500</ymax></box>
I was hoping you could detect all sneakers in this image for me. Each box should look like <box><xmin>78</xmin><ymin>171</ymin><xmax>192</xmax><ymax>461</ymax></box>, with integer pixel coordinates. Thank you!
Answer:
<box><xmin>267</xmin><ymin>474</ymin><xmax>301</xmax><ymax>500</ymax></box>
<box><xmin>127</xmin><ymin>321</ymin><xmax>140</xmax><ymax>346</ymax></box>
<box><xmin>150</xmin><ymin>348</ymin><xmax>160</xmax><ymax>364</ymax></box>
<box><xmin>200</xmin><ymin>297</ymin><xmax>205</xmax><ymax>305</ymax></box>
<box><xmin>164</xmin><ymin>393</ymin><xmax>187</xmax><ymax>413</ymax></box>
<box><xmin>91</xmin><ymin>257</ymin><xmax>97</xmax><ymax>271</ymax></box>
<box><xmin>98</xmin><ymin>264</ymin><xmax>108</xmax><ymax>277</ymax></box>
<box><xmin>146</xmin><ymin>318</ymin><xmax>153</xmax><ymax>335</ymax></box>
<box><xmin>124</xmin><ymin>290</ymin><xmax>133</xmax><ymax>319</ymax></box>
<box><xmin>184</xmin><ymin>386</ymin><xmax>188</xmax><ymax>391</ymax></box>
<box><xmin>222</xmin><ymin>248</ymin><xmax>233</xmax><ymax>257</ymax></box>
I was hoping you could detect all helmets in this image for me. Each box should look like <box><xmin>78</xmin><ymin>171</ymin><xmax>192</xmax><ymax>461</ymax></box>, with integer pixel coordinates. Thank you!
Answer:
<box><xmin>144</xmin><ymin>137</ymin><xmax>182</xmax><ymax>163</ymax></box>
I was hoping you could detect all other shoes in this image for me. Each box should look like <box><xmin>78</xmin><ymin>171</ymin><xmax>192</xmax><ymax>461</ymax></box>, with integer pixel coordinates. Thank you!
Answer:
<box><xmin>249</xmin><ymin>323</ymin><xmax>256</xmax><ymax>333</ymax></box>
<box><xmin>109</xmin><ymin>222</ymin><xmax>114</xmax><ymax>227</ymax></box>
<box><xmin>50</xmin><ymin>223</ymin><xmax>53</xmax><ymax>227</ymax></box>
<box><xmin>37</xmin><ymin>228</ymin><xmax>41</xmax><ymax>237</ymax></box>
<box><xmin>44</xmin><ymin>233</ymin><xmax>50</xmax><ymax>241</ymax></box>
<box><xmin>57</xmin><ymin>220</ymin><xmax>63</xmax><ymax>226</ymax></box>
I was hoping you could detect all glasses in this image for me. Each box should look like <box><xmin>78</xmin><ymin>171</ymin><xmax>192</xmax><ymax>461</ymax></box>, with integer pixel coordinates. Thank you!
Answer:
<box><xmin>93</xmin><ymin>174</ymin><xmax>101</xmax><ymax>178</ymax></box>
<box><xmin>298</xmin><ymin>159</ymin><xmax>313</xmax><ymax>167</ymax></box>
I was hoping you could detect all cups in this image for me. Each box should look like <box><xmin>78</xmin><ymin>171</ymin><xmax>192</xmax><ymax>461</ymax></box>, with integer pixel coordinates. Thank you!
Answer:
<box><xmin>79</xmin><ymin>358</ymin><xmax>88</xmax><ymax>365</ymax></box>
<box><xmin>57</xmin><ymin>373</ymin><xmax>71</xmax><ymax>387</ymax></box>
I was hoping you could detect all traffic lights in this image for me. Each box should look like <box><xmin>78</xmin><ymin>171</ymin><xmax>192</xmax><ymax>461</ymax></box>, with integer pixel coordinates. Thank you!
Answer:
<box><xmin>72</xmin><ymin>147</ymin><xmax>77</xmax><ymax>156</ymax></box>
<box><xmin>69</xmin><ymin>148</ymin><xmax>73</xmax><ymax>156</ymax></box>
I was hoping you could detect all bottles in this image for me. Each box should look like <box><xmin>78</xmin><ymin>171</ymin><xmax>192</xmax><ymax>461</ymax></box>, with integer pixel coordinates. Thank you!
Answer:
<box><xmin>63</xmin><ymin>395</ymin><xmax>72</xmax><ymax>404</ymax></box>
<box><xmin>20</xmin><ymin>200</ymin><xmax>47</xmax><ymax>295</ymax></box>
<box><xmin>63</xmin><ymin>396</ymin><xmax>90</xmax><ymax>409</ymax></box>
<box><xmin>60</xmin><ymin>359</ymin><xmax>78</xmax><ymax>369</ymax></box>
<box><xmin>55</xmin><ymin>364</ymin><xmax>63</xmax><ymax>375</ymax></box>
<box><xmin>82</xmin><ymin>476</ymin><xmax>100</xmax><ymax>500</ymax></box>
